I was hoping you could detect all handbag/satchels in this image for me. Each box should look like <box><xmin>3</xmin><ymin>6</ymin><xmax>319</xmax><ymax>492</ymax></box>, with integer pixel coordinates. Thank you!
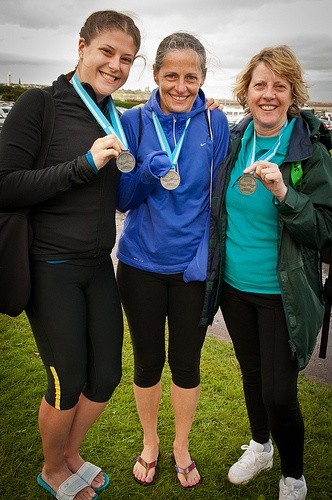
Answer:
<box><xmin>0</xmin><ymin>209</ymin><xmax>34</xmax><ymax>318</ymax></box>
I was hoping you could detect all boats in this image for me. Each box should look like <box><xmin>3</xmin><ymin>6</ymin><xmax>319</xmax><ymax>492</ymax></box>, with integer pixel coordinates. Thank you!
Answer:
<box><xmin>0</xmin><ymin>101</ymin><xmax>15</xmax><ymax>130</ymax></box>
<box><xmin>224</xmin><ymin>108</ymin><xmax>245</xmax><ymax>125</ymax></box>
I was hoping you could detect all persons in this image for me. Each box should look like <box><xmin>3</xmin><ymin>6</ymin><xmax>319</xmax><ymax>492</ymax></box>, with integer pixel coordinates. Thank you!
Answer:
<box><xmin>116</xmin><ymin>33</ymin><xmax>230</xmax><ymax>488</ymax></box>
<box><xmin>0</xmin><ymin>10</ymin><xmax>224</xmax><ymax>500</ymax></box>
<box><xmin>198</xmin><ymin>45</ymin><xmax>332</xmax><ymax>500</ymax></box>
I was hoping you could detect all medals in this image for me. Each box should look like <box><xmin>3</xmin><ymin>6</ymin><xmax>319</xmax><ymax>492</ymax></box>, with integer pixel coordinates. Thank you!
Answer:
<box><xmin>116</xmin><ymin>152</ymin><xmax>135</xmax><ymax>173</ymax></box>
<box><xmin>238</xmin><ymin>175</ymin><xmax>257</xmax><ymax>196</ymax></box>
<box><xmin>160</xmin><ymin>169</ymin><xmax>180</xmax><ymax>190</ymax></box>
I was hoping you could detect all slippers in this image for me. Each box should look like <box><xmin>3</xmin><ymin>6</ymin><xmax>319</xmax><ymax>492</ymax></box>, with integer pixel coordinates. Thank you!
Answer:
<box><xmin>73</xmin><ymin>461</ymin><xmax>110</xmax><ymax>491</ymax></box>
<box><xmin>171</xmin><ymin>450</ymin><xmax>202</xmax><ymax>489</ymax></box>
<box><xmin>36</xmin><ymin>469</ymin><xmax>98</xmax><ymax>500</ymax></box>
<box><xmin>132</xmin><ymin>450</ymin><xmax>161</xmax><ymax>486</ymax></box>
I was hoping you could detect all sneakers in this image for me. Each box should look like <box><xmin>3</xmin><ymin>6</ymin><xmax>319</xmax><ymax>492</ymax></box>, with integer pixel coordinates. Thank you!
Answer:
<box><xmin>228</xmin><ymin>439</ymin><xmax>274</xmax><ymax>486</ymax></box>
<box><xmin>278</xmin><ymin>474</ymin><xmax>308</xmax><ymax>500</ymax></box>
